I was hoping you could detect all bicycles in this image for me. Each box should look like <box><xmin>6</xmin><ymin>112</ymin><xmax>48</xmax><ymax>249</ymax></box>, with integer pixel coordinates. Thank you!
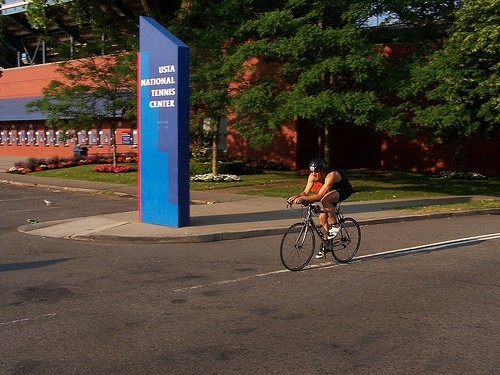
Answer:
<box><xmin>279</xmin><ymin>196</ymin><xmax>361</xmax><ymax>272</ymax></box>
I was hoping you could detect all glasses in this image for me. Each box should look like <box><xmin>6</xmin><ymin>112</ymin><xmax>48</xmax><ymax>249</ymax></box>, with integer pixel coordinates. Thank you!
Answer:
<box><xmin>311</xmin><ymin>171</ymin><xmax>318</xmax><ymax>173</ymax></box>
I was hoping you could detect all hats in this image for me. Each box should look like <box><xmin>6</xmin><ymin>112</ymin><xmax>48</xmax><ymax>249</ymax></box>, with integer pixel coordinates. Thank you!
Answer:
<box><xmin>309</xmin><ymin>159</ymin><xmax>328</xmax><ymax>171</ymax></box>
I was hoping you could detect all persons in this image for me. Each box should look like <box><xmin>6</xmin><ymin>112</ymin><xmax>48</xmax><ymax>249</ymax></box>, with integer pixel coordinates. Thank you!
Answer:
<box><xmin>287</xmin><ymin>159</ymin><xmax>353</xmax><ymax>259</ymax></box>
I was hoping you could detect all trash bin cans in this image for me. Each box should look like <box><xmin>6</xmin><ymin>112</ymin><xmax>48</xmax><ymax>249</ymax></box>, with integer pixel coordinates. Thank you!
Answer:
<box><xmin>73</xmin><ymin>146</ymin><xmax>88</xmax><ymax>158</ymax></box>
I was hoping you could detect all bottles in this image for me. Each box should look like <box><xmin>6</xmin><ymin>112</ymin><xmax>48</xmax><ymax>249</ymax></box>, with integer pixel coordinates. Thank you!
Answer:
<box><xmin>317</xmin><ymin>225</ymin><xmax>328</xmax><ymax>237</ymax></box>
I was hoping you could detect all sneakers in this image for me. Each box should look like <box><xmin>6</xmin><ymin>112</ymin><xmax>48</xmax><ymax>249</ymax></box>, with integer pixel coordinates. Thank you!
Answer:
<box><xmin>315</xmin><ymin>245</ymin><xmax>330</xmax><ymax>259</ymax></box>
<box><xmin>327</xmin><ymin>223</ymin><xmax>342</xmax><ymax>240</ymax></box>
